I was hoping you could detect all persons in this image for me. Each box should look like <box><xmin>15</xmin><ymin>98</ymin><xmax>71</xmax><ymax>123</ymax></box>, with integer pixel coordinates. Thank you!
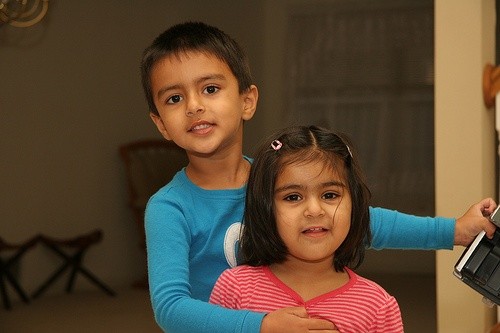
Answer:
<box><xmin>139</xmin><ymin>15</ymin><xmax>497</xmax><ymax>333</ymax></box>
<box><xmin>208</xmin><ymin>122</ymin><xmax>404</xmax><ymax>333</ymax></box>
